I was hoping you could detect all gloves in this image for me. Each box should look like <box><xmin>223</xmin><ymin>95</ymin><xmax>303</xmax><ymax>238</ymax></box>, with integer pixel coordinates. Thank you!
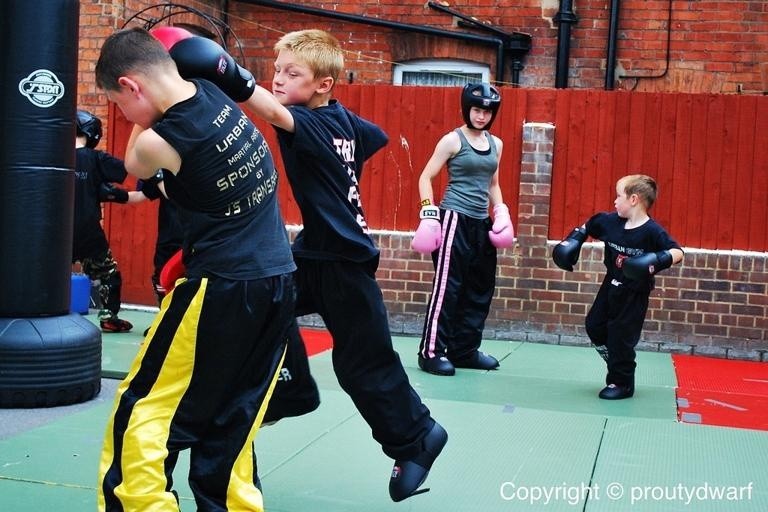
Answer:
<box><xmin>552</xmin><ymin>225</ymin><xmax>586</xmax><ymax>272</ymax></box>
<box><xmin>168</xmin><ymin>36</ymin><xmax>256</xmax><ymax>105</ymax></box>
<box><xmin>622</xmin><ymin>250</ymin><xmax>673</xmax><ymax>283</ymax></box>
<box><xmin>487</xmin><ymin>203</ymin><xmax>515</xmax><ymax>248</ymax></box>
<box><xmin>409</xmin><ymin>205</ymin><xmax>442</xmax><ymax>252</ymax></box>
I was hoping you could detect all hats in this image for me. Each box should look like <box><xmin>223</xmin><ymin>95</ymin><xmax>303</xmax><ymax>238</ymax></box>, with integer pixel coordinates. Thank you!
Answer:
<box><xmin>77</xmin><ymin>110</ymin><xmax>103</xmax><ymax>148</ymax></box>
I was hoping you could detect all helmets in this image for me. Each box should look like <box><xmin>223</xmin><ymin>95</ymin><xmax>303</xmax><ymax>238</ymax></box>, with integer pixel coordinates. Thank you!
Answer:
<box><xmin>461</xmin><ymin>82</ymin><xmax>501</xmax><ymax>111</ymax></box>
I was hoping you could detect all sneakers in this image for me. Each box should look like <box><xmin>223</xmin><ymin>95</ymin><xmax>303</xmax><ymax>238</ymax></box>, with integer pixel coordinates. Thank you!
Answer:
<box><xmin>389</xmin><ymin>421</ymin><xmax>449</xmax><ymax>504</ymax></box>
<box><xmin>417</xmin><ymin>353</ymin><xmax>455</xmax><ymax>376</ymax></box>
<box><xmin>598</xmin><ymin>381</ymin><xmax>635</xmax><ymax>400</ymax></box>
<box><xmin>259</xmin><ymin>376</ymin><xmax>321</xmax><ymax>426</ymax></box>
<box><xmin>449</xmin><ymin>351</ymin><xmax>500</xmax><ymax>370</ymax></box>
<box><xmin>100</xmin><ymin>318</ymin><xmax>134</xmax><ymax>332</ymax></box>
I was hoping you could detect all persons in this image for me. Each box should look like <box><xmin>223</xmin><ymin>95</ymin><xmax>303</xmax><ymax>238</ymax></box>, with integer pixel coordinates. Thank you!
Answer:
<box><xmin>93</xmin><ymin>27</ymin><xmax>297</xmax><ymax>511</ymax></box>
<box><xmin>150</xmin><ymin>24</ymin><xmax>195</xmax><ymax>297</ymax></box>
<box><xmin>552</xmin><ymin>174</ymin><xmax>686</xmax><ymax>400</ymax></box>
<box><xmin>75</xmin><ymin>111</ymin><xmax>131</xmax><ymax>331</ymax></box>
<box><xmin>410</xmin><ymin>82</ymin><xmax>515</xmax><ymax>376</ymax></box>
<box><xmin>169</xmin><ymin>30</ymin><xmax>448</xmax><ymax>503</ymax></box>
<box><xmin>99</xmin><ymin>171</ymin><xmax>173</xmax><ymax>338</ymax></box>
